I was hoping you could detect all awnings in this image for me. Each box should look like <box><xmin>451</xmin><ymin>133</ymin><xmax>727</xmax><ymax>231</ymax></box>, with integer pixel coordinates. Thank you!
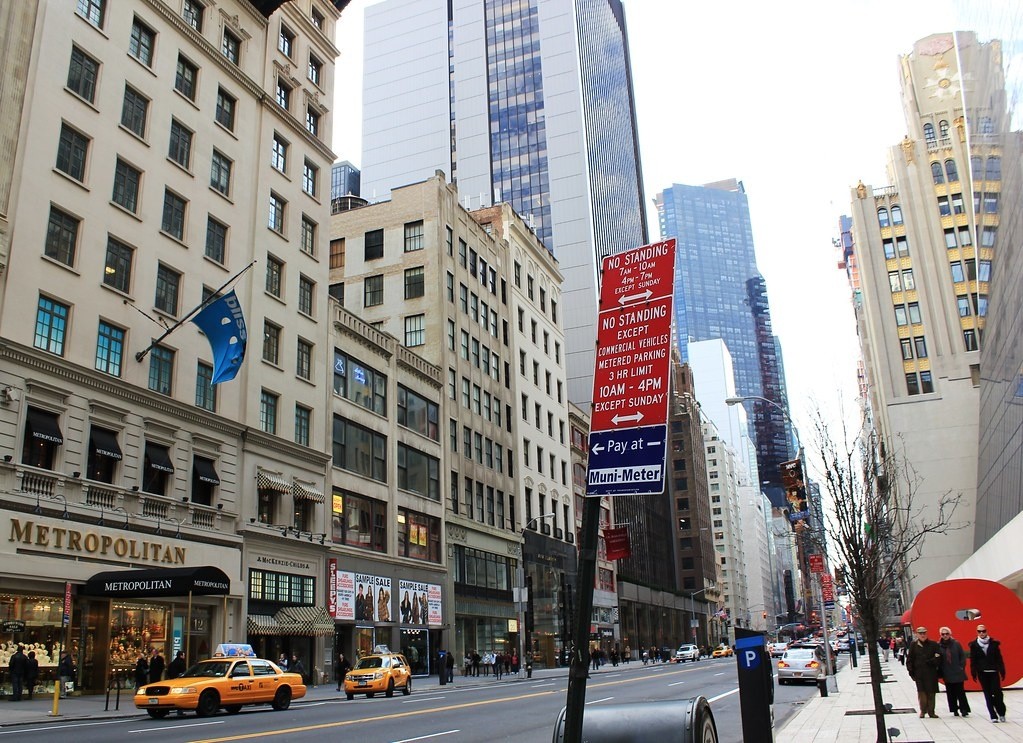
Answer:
<box><xmin>247</xmin><ymin>606</ymin><xmax>335</xmax><ymax>636</ymax></box>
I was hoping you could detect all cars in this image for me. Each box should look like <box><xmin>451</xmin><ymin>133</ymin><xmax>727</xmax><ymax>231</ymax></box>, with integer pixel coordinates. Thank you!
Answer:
<box><xmin>778</xmin><ymin>649</ymin><xmax>827</xmax><ymax>688</ymax></box>
<box><xmin>770</xmin><ymin>626</ymin><xmax>865</xmax><ymax>658</ymax></box>
<box><xmin>345</xmin><ymin>645</ymin><xmax>412</xmax><ymax>700</ymax></box>
<box><xmin>713</xmin><ymin>646</ymin><xmax>733</xmax><ymax>659</ymax></box>
<box><xmin>676</xmin><ymin>644</ymin><xmax>700</xmax><ymax>663</ymax></box>
<box><xmin>133</xmin><ymin>645</ymin><xmax>307</xmax><ymax>718</ymax></box>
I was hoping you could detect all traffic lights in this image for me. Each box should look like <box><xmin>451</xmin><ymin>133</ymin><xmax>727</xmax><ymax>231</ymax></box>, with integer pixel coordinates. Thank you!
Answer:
<box><xmin>763</xmin><ymin>612</ymin><xmax>766</xmax><ymax>619</ymax></box>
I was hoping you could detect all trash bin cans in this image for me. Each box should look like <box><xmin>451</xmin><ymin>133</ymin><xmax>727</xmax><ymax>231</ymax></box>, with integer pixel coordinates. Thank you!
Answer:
<box><xmin>662</xmin><ymin>651</ymin><xmax>671</xmax><ymax>663</ymax></box>
<box><xmin>858</xmin><ymin>642</ymin><xmax>865</xmax><ymax>655</ymax></box>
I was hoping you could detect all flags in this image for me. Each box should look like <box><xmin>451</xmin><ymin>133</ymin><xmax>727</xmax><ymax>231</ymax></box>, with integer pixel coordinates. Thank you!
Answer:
<box><xmin>189</xmin><ymin>289</ymin><xmax>247</xmax><ymax>386</ymax></box>
<box><xmin>602</xmin><ymin>526</ymin><xmax>632</xmax><ymax>560</ymax></box>
<box><xmin>714</xmin><ymin>609</ymin><xmax>724</xmax><ymax>617</ymax></box>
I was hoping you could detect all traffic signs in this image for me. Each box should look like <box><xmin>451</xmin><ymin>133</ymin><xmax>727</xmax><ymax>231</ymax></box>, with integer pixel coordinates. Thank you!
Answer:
<box><xmin>584</xmin><ymin>238</ymin><xmax>677</xmax><ymax>498</ymax></box>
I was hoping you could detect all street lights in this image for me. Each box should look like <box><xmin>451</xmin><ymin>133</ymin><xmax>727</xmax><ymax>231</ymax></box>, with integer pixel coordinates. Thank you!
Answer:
<box><xmin>726</xmin><ymin>395</ymin><xmax>837</xmax><ymax>694</ymax></box>
<box><xmin>746</xmin><ymin>603</ymin><xmax>764</xmax><ymax>629</ymax></box>
<box><xmin>691</xmin><ymin>586</ymin><xmax>715</xmax><ymax>645</ymax></box>
<box><xmin>519</xmin><ymin>513</ymin><xmax>557</xmax><ymax>679</ymax></box>
<box><xmin>774</xmin><ymin>612</ymin><xmax>789</xmax><ymax>629</ymax></box>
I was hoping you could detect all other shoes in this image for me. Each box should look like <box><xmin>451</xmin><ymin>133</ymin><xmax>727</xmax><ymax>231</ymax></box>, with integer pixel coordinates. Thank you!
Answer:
<box><xmin>992</xmin><ymin>719</ymin><xmax>998</xmax><ymax>723</ymax></box>
<box><xmin>920</xmin><ymin>711</ymin><xmax>924</xmax><ymax>718</ymax></box>
<box><xmin>336</xmin><ymin>688</ymin><xmax>340</xmax><ymax>692</ymax></box>
<box><xmin>929</xmin><ymin>712</ymin><xmax>938</xmax><ymax>718</ymax></box>
<box><xmin>954</xmin><ymin>711</ymin><xmax>959</xmax><ymax>716</ymax></box>
<box><xmin>962</xmin><ymin>711</ymin><xmax>968</xmax><ymax>716</ymax></box>
<box><xmin>1000</xmin><ymin>716</ymin><xmax>1005</xmax><ymax>722</ymax></box>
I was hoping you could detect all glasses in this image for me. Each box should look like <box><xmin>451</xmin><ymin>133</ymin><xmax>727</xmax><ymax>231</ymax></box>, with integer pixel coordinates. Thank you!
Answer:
<box><xmin>978</xmin><ymin>630</ymin><xmax>986</xmax><ymax>633</ymax></box>
<box><xmin>942</xmin><ymin>633</ymin><xmax>949</xmax><ymax>636</ymax></box>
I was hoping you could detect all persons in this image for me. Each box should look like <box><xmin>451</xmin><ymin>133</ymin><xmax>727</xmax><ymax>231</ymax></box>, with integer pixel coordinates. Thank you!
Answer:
<box><xmin>173</xmin><ymin>651</ymin><xmax>187</xmax><ymax>715</ymax></box>
<box><xmin>464</xmin><ymin>647</ymin><xmax>533</xmax><ymax>678</ymax></box>
<box><xmin>356</xmin><ymin>583</ymin><xmax>373</xmax><ymax>620</ymax></box>
<box><xmin>355</xmin><ymin>648</ymin><xmax>363</xmax><ymax>664</ymax></box>
<box><xmin>401</xmin><ymin>591</ymin><xmax>428</xmax><ymax>625</ymax></box>
<box><xmin>641</xmin><ymin>645</ymin><xmax>661</xmax><ymax>665</ymax></box>
<box><xmin>150</xmin><ymin>649</ymin><xmax>164</xmax><ymax>684</ymax></box>
<box><xmin>878</xmin><ymin>633</ymin><xmax>907</xmax><ymax>665</ymax></box>
<box><xmin>591</xmin><ymin>646</ymin><xmax>631</xmax><ymax>670</ymax></box>
<box><xmin>60</xmin><ymin>651</ymin><xmax>73</xmax><ymax>699</ymax></box>
<box><xmin>906</xmin><ymin>625</ymin><xmax>1006</xmax><ymax>722</ymax></box>
<box><xmin>446</xmin><ymin>652</ymin><xmax>454</xmax><ymax>683</ymax></box>
<box><xmin>135</xmin><ymin>652</ymin><xmax>148</xmax><ymax>695</ymax></box>
<box><xmin>336</xmin><ymin>653</ymin><xmax>352</xmax><ymax>691</ymax></box>
<box><xmin>288</xmin><ymin>655</ymin><xmax>308</xmax><ymax>675</ymax></box>
<box><xmin>406</xmin><ymin>644</ymin><xmax>419</xmax><ymax>675</ymax></box>
<box><xmin>378</xmin><ymin>587</ymin><xmax>390</xmax><ymax>621</ymax></box>
<box><xmin>9</xmin><ymin>645</ymin><xmax>28</xmax><ymax>701</ymax></box>
<box><xmin>23</xmin><ymin>651</ymin><xmax>38</xmax><ymax>700</ymax></box>
<box><xmin>700</xmin><ymin>644</ymin><xmax>713</xmax><ymax>658</ymax></box>
<box><xmin>279</xmin><ymin>653</ymin><xmax>287</xmax><ymax>672</ymax></box>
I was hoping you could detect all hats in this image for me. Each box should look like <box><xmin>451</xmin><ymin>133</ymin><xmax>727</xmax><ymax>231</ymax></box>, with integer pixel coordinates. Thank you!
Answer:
<box><xmin>917</xmin><ymin>627</ymin><xmax>927</xmax><ymax>633</ymax></box>
